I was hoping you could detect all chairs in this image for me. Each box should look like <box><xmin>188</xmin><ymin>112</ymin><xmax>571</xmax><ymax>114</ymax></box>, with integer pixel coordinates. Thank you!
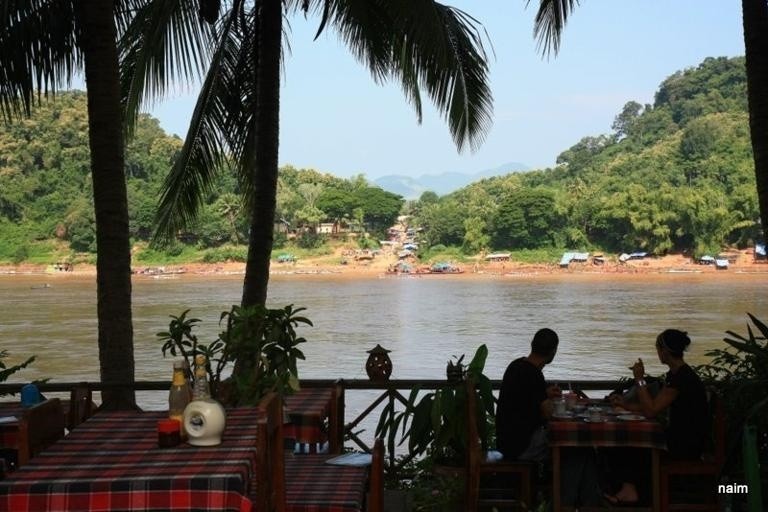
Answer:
<box><xmin>16</xmin><ymin>379</ymin><xmax>384</xmax><ymax>512</ymax></box>
<box><xmin>465</xmin><ymin>383</ymin><xmax>538</xmax><ymax>512</ymax></box>
<box><xmin>660</xmin><ymin>398</ymin><xmax>725</xmax><ymax>512</ymax></box>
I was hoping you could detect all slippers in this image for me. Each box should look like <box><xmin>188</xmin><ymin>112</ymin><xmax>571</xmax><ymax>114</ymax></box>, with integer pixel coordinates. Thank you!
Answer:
<box><xmin>600</xmin><ymin>493</ymin><xmax>620</xmax><ymax>510</ymax></box>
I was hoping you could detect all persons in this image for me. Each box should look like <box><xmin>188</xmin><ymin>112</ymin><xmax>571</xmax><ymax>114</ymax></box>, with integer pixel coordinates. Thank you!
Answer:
<box><xmin>495</xmin><ymin>327</ymin><xmax>579</xmax><ymax>512</ymax></box>
<box><xmin>602</xmin><ymin>328</ymin><xmax>706</xmax><ymax>511</ymax></box>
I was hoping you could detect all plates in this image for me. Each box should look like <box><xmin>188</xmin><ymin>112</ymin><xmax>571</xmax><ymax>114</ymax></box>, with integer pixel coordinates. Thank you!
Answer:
<box><xmin>607</xmin><ymin>410</ymin><xmax>632</xmax><ymax>414</ymax></box>
<box><xmin>583</xmin><ymin>418</ymin><xmax>608</xmax><ymax>423</ymax></box>
<box><xmin>616</xmin><ymin>414</ymin><xmax>645</xmax><ymax>421</ymax></box>
<box><xmin>552</xmin><ymin>410</ymin><xmax>578</xmax><ymax>419</ymax></box>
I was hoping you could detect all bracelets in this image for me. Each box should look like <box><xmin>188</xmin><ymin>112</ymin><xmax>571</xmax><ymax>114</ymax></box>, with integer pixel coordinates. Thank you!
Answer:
<box><xmin>636</xmin><ymin>378</ymin><xmax>647</xmax><ymax>388</ymax></box>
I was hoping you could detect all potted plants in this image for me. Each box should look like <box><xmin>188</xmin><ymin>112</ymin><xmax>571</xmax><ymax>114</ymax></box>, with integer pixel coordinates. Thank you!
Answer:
<box><xmin>383</xmin><ymin>466</ymin><xmax>407</xmax><ymax>512</ymax></box>
<box><xmin>399</xmin><ymin>345</ymin><xmax>496</xmax><ymax>502</ymax></box>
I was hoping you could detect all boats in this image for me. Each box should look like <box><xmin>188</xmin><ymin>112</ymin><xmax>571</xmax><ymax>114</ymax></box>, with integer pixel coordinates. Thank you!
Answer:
<box><xmin>385</xmin><ymin>259</ymin><xmax>432</xmax><ymax>275</ymax></box>
<box><xmin>421</xmin><ymin>263</ymin><xmax>464</xmax><ymax>274</ymax></box>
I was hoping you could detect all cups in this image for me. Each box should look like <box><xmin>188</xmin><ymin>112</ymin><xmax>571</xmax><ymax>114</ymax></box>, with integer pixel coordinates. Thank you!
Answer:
<box><xmin>553</xmin><ymin>401</ymin><xmax>565</xmax><ymax>416</ymax></box>
<box><xmin>564</xmin><ymin>393</ymin><xmax>578</xmax><ymax>410</ymax></box>
<box><xmin>584</xmin><ymin>407</ymin><xmax>602</xmax><ymax>419</ymax></box>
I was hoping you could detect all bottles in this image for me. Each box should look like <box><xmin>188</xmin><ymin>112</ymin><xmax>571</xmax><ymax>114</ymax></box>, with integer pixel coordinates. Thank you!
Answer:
<box><xmin>191</xmin><ymin>354</ymin><xmax>212</xmax><ymax>402</ymax></box>
<box><xmin>157</xmin><ymin>418</ymin><xmax>182</xmax><ymax>449</ymax></box>
<box><xmin>168</xmin><ymin>361</ymin><xmax>190</xmax><ymax>444</ymax></box>
